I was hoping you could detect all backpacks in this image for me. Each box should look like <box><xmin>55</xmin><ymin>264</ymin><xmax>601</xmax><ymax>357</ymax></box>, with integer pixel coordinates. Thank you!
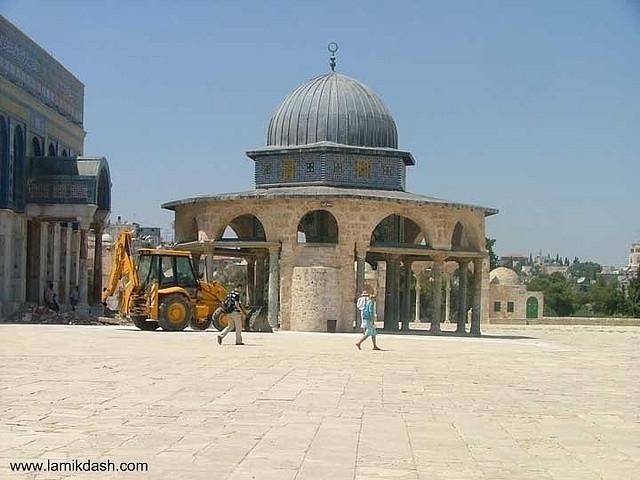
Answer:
<box><xmin>223</xmin><ymin>297</ymin><xmax>234</xmax><ymax>312</ymax></box>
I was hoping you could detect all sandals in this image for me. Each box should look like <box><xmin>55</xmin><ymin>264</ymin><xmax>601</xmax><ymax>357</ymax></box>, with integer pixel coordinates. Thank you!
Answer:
<box><xmin>373</xmin><ymin>347</ymin><xmax>380</xmax><ymax>350</ymax></box>
<box><xmin>356</xmin><ymin>343</ymin><xmax>361</xmax><ymax>350</ymax></box>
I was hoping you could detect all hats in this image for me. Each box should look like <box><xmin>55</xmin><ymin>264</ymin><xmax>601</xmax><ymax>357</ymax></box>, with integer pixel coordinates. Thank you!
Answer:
<box><xmin>362</xmin><ymin>291</ymin><xmax>368</xmax><ymax>296</ymax></box>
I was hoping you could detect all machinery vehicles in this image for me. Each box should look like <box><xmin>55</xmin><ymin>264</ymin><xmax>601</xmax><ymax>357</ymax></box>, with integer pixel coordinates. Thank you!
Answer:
<box><xmin>102</xmin><ymin>229</ymin><xmax>274</xmax><ymax>333</ymax></box>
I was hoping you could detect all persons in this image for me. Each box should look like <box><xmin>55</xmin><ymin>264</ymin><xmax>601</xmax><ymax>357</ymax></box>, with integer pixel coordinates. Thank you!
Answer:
<box><xmin>69</xmin><ymin>286</ymin><xmax>80</xmax><ymax>309</ymax></box>
<box><xmin>45</xmin><ymin>283</ymin><xmax>54</xmax><ymax>308</ymax></box>
<box><xmin>102</xmin><ymin>288</ymin><xmax>108</xmax><ymax>317</ymax></box>
<box><xmin>218</xmin><ymin>283</ymin><xmax>247</xmax><ymax>345</ymax></box>
<box><xmin>355</xmin><ymin>290</ymin><xmax>381</xmax><ymax>350</ymax></box>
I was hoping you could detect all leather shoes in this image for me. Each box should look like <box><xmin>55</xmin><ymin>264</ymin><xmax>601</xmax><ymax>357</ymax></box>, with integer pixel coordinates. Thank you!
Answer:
<box><xmin>218</xmin><ymin>336</ymin><xmax>222</xmax><ymax>344</ymax></box>
<box><xmin>236</xmin><ymin>343</ymin><xmax>244</xmax><ymax>345</ymax></box>
